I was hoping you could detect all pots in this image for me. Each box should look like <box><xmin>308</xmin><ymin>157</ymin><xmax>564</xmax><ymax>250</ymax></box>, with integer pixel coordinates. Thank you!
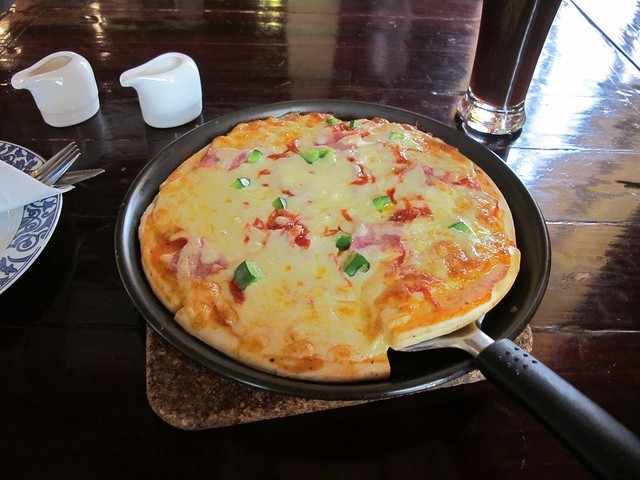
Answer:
<box><xmin>108</xmin><ymin>92</ymin><xmax>640</xmax><ymax>480</ymax></box>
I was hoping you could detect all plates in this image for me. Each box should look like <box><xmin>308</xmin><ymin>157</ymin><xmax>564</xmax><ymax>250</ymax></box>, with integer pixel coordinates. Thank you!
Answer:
<box><xmin>0</xmin><ymin>138</ymin><xmax>64</xmax><ymax>293</ymax></box>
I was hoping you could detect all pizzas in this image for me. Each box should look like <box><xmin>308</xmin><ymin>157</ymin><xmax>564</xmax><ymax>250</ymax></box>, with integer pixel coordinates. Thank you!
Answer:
<box><xmin>139</xmin><ymin>111</ymin><xmax>522</xmax><ymax>382</ymax></box>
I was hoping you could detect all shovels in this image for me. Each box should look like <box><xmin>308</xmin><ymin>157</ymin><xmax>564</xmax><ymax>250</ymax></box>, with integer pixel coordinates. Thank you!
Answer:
<box><xmin>401</xmin><ymin>311</ymin><xmax>640</xmax><ymax>480</ymax></box>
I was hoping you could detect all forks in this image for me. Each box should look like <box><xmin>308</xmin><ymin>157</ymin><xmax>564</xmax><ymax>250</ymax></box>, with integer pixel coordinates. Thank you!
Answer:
<box><xmin>30</xmin><ymin>139</ymin><xmax>85</xmax><ymax>184</ymax></box>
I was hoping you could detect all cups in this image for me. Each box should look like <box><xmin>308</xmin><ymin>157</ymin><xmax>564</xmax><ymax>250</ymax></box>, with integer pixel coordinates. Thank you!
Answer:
<box><xmin>117</xmin><ymin>51</ymin><xmax>206</xmax><ymax>128</ymax></box>
<box><xmin>10</xmin><ymin>48</ymin><xmax>103</xmax><ymax>128</ymax></box>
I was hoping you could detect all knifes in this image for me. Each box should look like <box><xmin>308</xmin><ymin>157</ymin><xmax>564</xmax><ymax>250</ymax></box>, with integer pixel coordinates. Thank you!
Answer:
<box><xmin>60</xmin><ymin>165</ymin><xmax>106</xmax><ymax>186</ymax></box>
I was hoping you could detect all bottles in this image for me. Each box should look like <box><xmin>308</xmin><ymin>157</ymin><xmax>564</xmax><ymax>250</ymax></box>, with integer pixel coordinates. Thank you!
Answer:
<box><xmin>456</xmin><ymin>0</ymin><xmax>563</xmax><ymax>138</ymax></box>
